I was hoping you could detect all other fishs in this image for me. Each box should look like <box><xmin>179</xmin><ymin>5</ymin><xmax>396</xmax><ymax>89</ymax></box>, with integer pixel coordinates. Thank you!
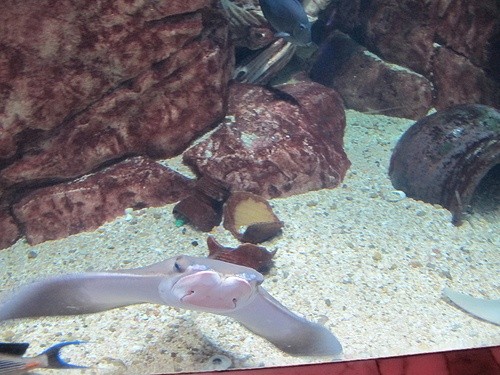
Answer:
<box><xmin>0</xmin><ymin>341</ymin><xmax>92</xmax><ymax>375</ymax></box>
<box><xmin>217</xmin><ymin>0</ymin><xmax>273</xmax><ymax>50</ymax></box>
<box><xmin>260</xmin><ymin>0</ymin><xmax>313</xmax><ymax>47</ymax></box>
<box><xmin>0</xmin><ymin>255</ymin><xmax>343</xmax><ymax>357</ymax></box>
